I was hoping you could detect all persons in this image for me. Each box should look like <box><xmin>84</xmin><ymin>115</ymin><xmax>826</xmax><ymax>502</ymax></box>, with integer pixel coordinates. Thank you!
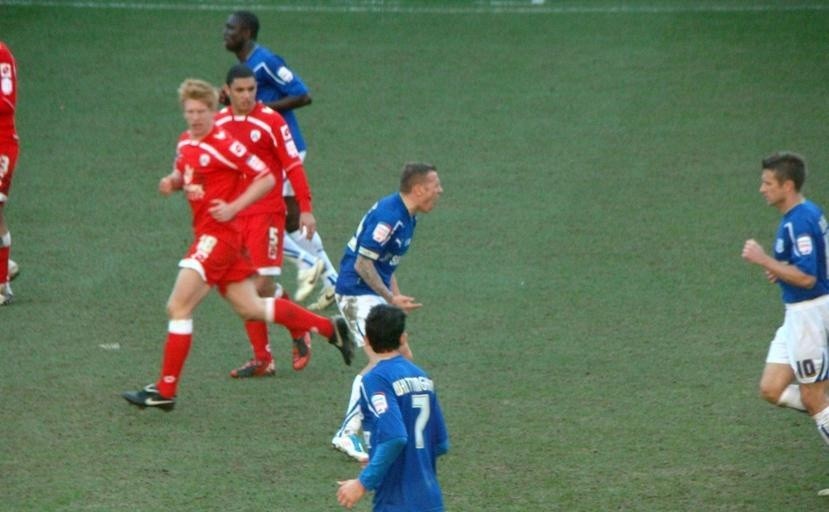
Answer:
<box><xmin>221</xmin><ymin>11</ymin><xmax>340</xmax><ymax>309</ymax></box>
<box><xmin>0</xmin><ymin>42</ymin><xmax>21</xmax><ymax>306</ymax></box>
<box><xmin>330</xmin><ymin>163</ymin><xmax>442</xmax><ymax>461</ymax></box>
<box><xmin>742</xmin><ymin>152</ymin><xmax>828</xmax><ymax>498</ymax></box>
<box><xmin>336</xmin><ymin>305</ymin><xmax>447</xmax><ymax>511</ymax></box>
<box><xmin>215</xmin><ymin>68</ymin><xmax>316</xmax><ymax>380</ymax></box>
<box><xmin>121</xmin><ymin>76</ymin><xmax>356</xmax><ymax>412</ymax></box>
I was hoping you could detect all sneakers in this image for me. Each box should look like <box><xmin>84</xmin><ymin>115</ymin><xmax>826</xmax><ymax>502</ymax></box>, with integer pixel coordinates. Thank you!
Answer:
<box><xmin>328</xmin><ymin>314</ymin><xmax>355</xmax><ymax>365</ymax></box>
<box><xmin>292</xmin><ymin>330</ymin><xmax>312</xmax><ymax>371</ymax></box>
<box><xmin>122</xmin><ymin>384</ymin><xmax>175</xmax><ymax>411</ymax></box>
<box><xmin>304</xmin><ymin>283</ymin><xmax>336</xmax><ymax>312</ymax></box>
<box><xmin>0</xmin><ymin>260</ymin><xmax>21</xmax><ymax>305</ymax></box>
<box><xmin>231</xmin><ymin>357</ymin><xmax>276</xmax><ymax>378</ymax></box>
<box><xmin>293</xmin><ymin>259</ymin><xmax>326</xmax><ymax>304</ymax></box>
<box><xmin>330</xmin><ymin>432</ymin><xmax>369</xmax><ymax>463</ymax></box>
